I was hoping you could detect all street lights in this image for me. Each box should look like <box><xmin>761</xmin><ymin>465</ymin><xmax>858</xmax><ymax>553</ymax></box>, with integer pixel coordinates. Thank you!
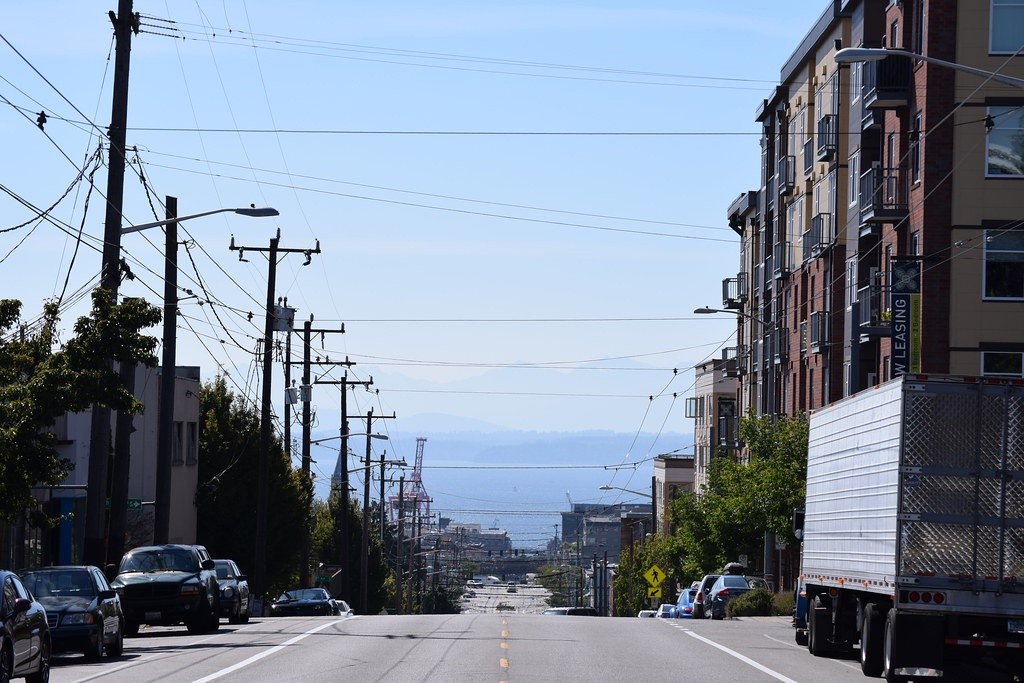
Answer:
<box><xmin>81</xmin><ymin>203</ymin><xmax>282</xmax><ymax>566</ymax></box>
<box><xmin>301</xmin><ymin>433</ymin><xmax>390</xmax><ymax>586</ymax></box>
<box><xmin>340</xmin><ymin>461</ymin><xmax>409</xmax><ymax>574</ymax></box>
<box><xmin>694</xmin><ymin>307</ymin><xmax>775</xmax><ymax>584</ymax></box>
<box><xmin>599</xmin><ymin>475</ymin><xmax>658</xmax><ymax>543</ymax></box>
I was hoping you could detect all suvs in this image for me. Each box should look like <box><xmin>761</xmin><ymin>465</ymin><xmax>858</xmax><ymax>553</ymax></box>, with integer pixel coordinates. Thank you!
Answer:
<box><xmin>108</xmin><ymin>543</ymin><xmax>220</xmax><ymax>638</ymax></box>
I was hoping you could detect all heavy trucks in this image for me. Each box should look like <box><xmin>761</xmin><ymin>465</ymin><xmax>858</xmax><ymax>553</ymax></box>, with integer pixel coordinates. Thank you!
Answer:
<box><xmin>792</xmin><ymin>371</ymin><xmax>1024</xmax><ymax>683</ymax></box>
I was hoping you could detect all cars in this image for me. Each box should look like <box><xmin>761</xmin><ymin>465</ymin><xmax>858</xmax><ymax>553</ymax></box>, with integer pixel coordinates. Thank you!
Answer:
<box><xmin>11</xmin><ymin>565</ymin><xmax>126</xmax><ymax>662</ymax></box>
<box><xmin>507</xmin><ymin>581</ymin><xmax>515</xmax><ymax>586</ymax></box>
<box><xmin>0</xmin><ymin>568</ymin><xmax>52</xmax><ymax>683</ymax></box>
<box><xmin>506</xmin><ymin>585</ymin><xmax>517</xmax><ymax>593</ymax></box>
<box><xmin>655</xmin><ymin>604</ymin><xmax>677</xmax><ymax>618</ymax></box>
<box><xmin>211</xmin><ymin>559</ymin><xmax>250</xmax><ymax>623</ymax></box>
<box><xmin>702</xmin><ymin>573</ymin><xmax>772</xmax><ymax>620</ymax></box>
<box><xmin>269</xmin><ymin>588</ymin><xmax>335</xmax><ymax>618</ymax></box>
<box><xmin>542</xmin><ymin>607</ymin><xmax>574</xmax><ymax>616</ymax></box>
<box><xmin>669</xmin><ymin>574</ymin><xmax>725</xmax><ymax>619</ymax></box>
<box><xmin>565</xmin><ymin>607</ymin><xmax>599</xmax><ymax>617</ymax></box>
<box><xmin>329</xmin><ymin>599</ymin><xmax>355</xmax><ymax>616</ymax></box>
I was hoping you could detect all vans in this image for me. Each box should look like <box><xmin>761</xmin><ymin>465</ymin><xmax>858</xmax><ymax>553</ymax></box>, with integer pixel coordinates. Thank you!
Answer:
<box><xmin>637</xmin><ymin>610</ymin><xmax>657</xmax><ymax>619</ymax></box>
